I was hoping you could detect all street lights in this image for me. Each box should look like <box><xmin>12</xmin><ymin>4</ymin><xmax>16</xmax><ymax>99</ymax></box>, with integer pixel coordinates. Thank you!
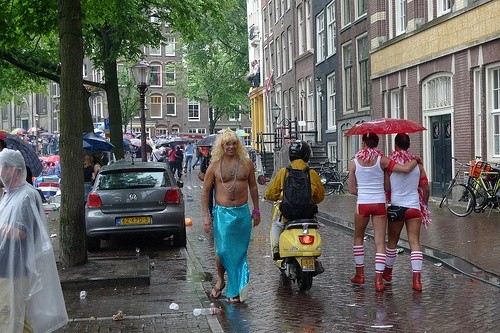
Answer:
<box><xmin>167</xmin><ymin>120</ymin><xmax>171</xmax><ymax>139</ymax></box>
<box><xmin>130</xmin><ymin>58</ymin><xmax>151</xmax><ymax>162</ymax></box>
<box><xmin>271</xmin><ymin>102</ymin><xmax>282</xmax><ymax>148</ymax></box>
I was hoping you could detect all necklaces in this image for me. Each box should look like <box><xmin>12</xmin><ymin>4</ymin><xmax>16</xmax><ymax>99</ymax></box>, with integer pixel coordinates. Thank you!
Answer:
<box><xmin>218</xmin><ymin>154</ymin><xmax>237</xmax><ymax>191</ymax></box>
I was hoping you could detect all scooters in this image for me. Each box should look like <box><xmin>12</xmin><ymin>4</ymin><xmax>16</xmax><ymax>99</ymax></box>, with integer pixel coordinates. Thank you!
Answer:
<box><xmin>257</xmin><ymin>175</ymin><xmax>323</xmax><ymax>291</ymax></box>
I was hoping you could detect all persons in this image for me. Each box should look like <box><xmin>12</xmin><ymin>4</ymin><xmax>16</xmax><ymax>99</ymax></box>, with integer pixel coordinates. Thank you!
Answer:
<box><xmin>82</xmin><ymin>152</ymin><xmax>110</xmax><ymax>210</ymax></box>
<box><xmin>200</xmin><ymin>128</ymin><xmax>261</xmax><ymax>303</ymax></box>
<box><xmin>21</xmin><ymin>136</ymin><xmax>61</xmax><ymax>156</ymax></box>
<box><xmin>264</xmin><ymin>141</ymin><xmax>326</xmax><ymax>275</ymax></box>
<box><xmin>250</xmin><ymin>59</ymin><xmax>260</xmax><ymax>89</ymax></box>
<box><xmin>198</xmin><ymin>147</ymin><xmax>219</xmax><ymax>222</ymax></box>
<box><xmin>150</xmin><ymin>143</ymin><xmax>204</xmax><ymax>178</ymax></box>
<box><xmin>1</xmin><ymin>148</ymin><xmax>39</xmax><ymax>333</ymax></box>
<box><xmin>348</xmin><ymin>132</ymin><xmax>423</xmax><ymax>293</ymax></box>
<box><xmin>382</xmin><ymin>132</ymin><xmax>430</xmax><ymax>292</ymax></box>
<box><xmin>0</xmin><ymin>139</ymin><xmax>47</xmax><ymax>202</ymax></box>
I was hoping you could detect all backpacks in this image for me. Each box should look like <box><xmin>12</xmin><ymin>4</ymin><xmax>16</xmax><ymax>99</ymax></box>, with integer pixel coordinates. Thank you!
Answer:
<box><xmin>279</xmin><ymin>165</ymin><xmax>318</xmax><ymax>223</ymax></box>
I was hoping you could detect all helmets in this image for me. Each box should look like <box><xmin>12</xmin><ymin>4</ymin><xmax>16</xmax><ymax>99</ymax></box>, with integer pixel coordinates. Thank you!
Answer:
<box><xmin>288</xmin><ymin>140</ymin><xmax>311</xmax><ymax>163</ymax></box>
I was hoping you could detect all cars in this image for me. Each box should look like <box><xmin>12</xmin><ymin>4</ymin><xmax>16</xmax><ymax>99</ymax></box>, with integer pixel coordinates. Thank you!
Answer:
<box><xmin>84</xmin><ymin>161</ymin><xmax>187</xmax><ymax>252</ymax></box>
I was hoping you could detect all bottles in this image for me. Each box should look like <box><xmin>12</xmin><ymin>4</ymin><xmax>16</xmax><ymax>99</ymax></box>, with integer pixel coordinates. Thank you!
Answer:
<box><xmin>201</xmin><ymin>307</ymin><xmax>222</xmax><ymax>315</ymax></box>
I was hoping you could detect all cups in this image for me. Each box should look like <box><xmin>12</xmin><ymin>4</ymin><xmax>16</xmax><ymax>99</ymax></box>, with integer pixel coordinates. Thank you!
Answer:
<box><xmin>169</xmin><ymin>302</ymin><xmax>179</xmax><ymax>310</ymax></box>
<box><xmin>80</xmin><ymin>291</ymin><xmax>87</xmax><ymax>299</ymax></box>
<box><xmin>193</xmin><ymin>308</ymin><xmax>202</xmax><ymax>316</ymax></box>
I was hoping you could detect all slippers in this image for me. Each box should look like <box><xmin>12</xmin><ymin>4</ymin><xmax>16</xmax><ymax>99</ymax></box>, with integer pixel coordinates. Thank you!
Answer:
<box><xmin>209</xmin><ymin>282</ymin><xmax>226</xmax><ymax>298</ymax></box>
<box><xmin>230</xmin><ymin>296</ymin><xmax>240</xmax><ymax>306</ymax></box>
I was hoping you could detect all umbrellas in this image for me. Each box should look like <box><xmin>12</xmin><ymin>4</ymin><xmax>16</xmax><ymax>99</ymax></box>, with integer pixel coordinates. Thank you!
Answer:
<box><xmin>81</xmin><ymin>132</ymin><xmax>116</xmax><ymax>151</ymax></box>
<box><xmin>342</xmin><ymin>117</ymin><xmax>427</xmax><ymax>157</ymax></box>
<box><xmin>10</xmin><ymin>127</ymin><xmax>61</xmax><ymax>137</ymax></box>
<box><xmin>195</xmin><ymin>135</ymin><xmax>220</xmax><ymax>148</ymax></box>
<box><xmin>119</xmin><ymin>128</ymin><xmax>234</xmax><ymax>153</ymax></box>
<box><xmin>0</xmin><ymin>131</ymin><xmax>44</xmax><ymax>177</ymax></box>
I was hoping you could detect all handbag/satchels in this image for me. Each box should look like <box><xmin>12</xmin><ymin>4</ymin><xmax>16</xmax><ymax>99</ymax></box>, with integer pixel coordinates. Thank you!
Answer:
<box><xmin>387</xmin><ymin>206</ymin><xmax>408</xmax><ymax>222</ymax></box>
<box><xmin>198</xmin><ymin>171</ymin><xmax>205</xmax><ymax>181</ymax></box>
<box><xmin>158</xmin><ymin>157</ymin><xmax>164</xmax><ymax>162</ymax></box>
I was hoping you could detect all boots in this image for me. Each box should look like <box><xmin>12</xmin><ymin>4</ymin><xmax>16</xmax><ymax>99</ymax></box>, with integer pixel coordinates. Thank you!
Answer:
<box><xmin>383</xmin><ymin>267</ymin><xmax>393</xmax><ymax>282</ymax></box>
<box><xmin>350</xmin><ymin>266</ymin><xmax>365</xmax><ymax>285</ymax></box>
<box><xmin>412</xmin><ymin>272</ymin><xmax>422</xmax><ymax>291</ymax></box>
<box><xmin>374</xmin><ymin>273</ymin><xmax>385</xmax><ymax>292</ymax></box>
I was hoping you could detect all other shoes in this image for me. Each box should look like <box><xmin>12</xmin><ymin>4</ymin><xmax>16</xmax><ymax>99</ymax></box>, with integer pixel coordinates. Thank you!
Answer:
<box><xmin>273</xmin><ymin>253</ymin><xmax>280</xmax><ymax>260</ymax></box>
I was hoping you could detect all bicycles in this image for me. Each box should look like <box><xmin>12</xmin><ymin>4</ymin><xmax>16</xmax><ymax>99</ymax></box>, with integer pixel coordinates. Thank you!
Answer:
<box><xmin>312</xmin><ymin>157</ymin><xmax>357</xmax><ymax>196</ymax></box>
<box><xmin>439</xmin><ymin>155</ymin><xmax>500</xmax><ymax>217</ymax></box>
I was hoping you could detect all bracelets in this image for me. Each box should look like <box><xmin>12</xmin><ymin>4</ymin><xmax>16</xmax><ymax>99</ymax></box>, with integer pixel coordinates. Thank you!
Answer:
<box><xmin>414</xmin><ymin>157</ymin><xmax>423</xmax><ymax>166</ymax></box>
<box><xmin>252</xmin><ymin>209</ymin><xmax>261</xmax><ymax>216</ymax></box>
